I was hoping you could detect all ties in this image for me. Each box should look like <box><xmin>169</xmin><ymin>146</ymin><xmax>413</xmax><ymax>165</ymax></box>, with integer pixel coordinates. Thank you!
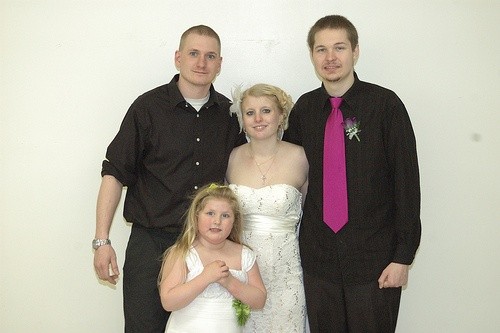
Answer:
<box><xmin>322</xmin><ymin>97</ymin><xmax>349</xmax><ymax>234</ymax></box>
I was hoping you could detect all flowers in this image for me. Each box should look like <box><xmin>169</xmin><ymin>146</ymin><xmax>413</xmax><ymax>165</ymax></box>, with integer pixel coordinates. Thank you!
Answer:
<box><xmin>341</xmin><ymin>117</ymin><xmax>362</xmax><ymax>141</ymax></box>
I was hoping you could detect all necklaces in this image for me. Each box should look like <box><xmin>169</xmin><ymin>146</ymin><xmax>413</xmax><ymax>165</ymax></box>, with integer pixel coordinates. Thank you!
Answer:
<box><xmin>248</xmin><ymin>141</ymin><xmax>279</xmax><ymax>181</ymax></box>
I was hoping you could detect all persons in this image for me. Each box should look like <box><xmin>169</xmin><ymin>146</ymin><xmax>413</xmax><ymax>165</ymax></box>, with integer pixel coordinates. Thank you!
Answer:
<box><xmin>157</xmin><ymin>182</ymin><xmax>267</xmax><ymax>333</ymax></box>
<box><xmin>92</xmin><ymin>24</ymin><xmax>249</xmax><ymax>333</ymax></box>
<box><xmin>282</xmin><ymin>15</ymin><xmax>422</xmax><ymax>333</ymax></box>
<box><xmin>225</xmin><ymin>83</ymin><xmax>310</xmax><ymax>333</ymax></box>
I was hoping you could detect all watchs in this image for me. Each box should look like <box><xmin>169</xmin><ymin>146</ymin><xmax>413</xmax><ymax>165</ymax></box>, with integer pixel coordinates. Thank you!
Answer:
<box><xmin>92</xmin><ymin>239</ymin><xmax>111</xmax><ymax>250</ymax></box>
